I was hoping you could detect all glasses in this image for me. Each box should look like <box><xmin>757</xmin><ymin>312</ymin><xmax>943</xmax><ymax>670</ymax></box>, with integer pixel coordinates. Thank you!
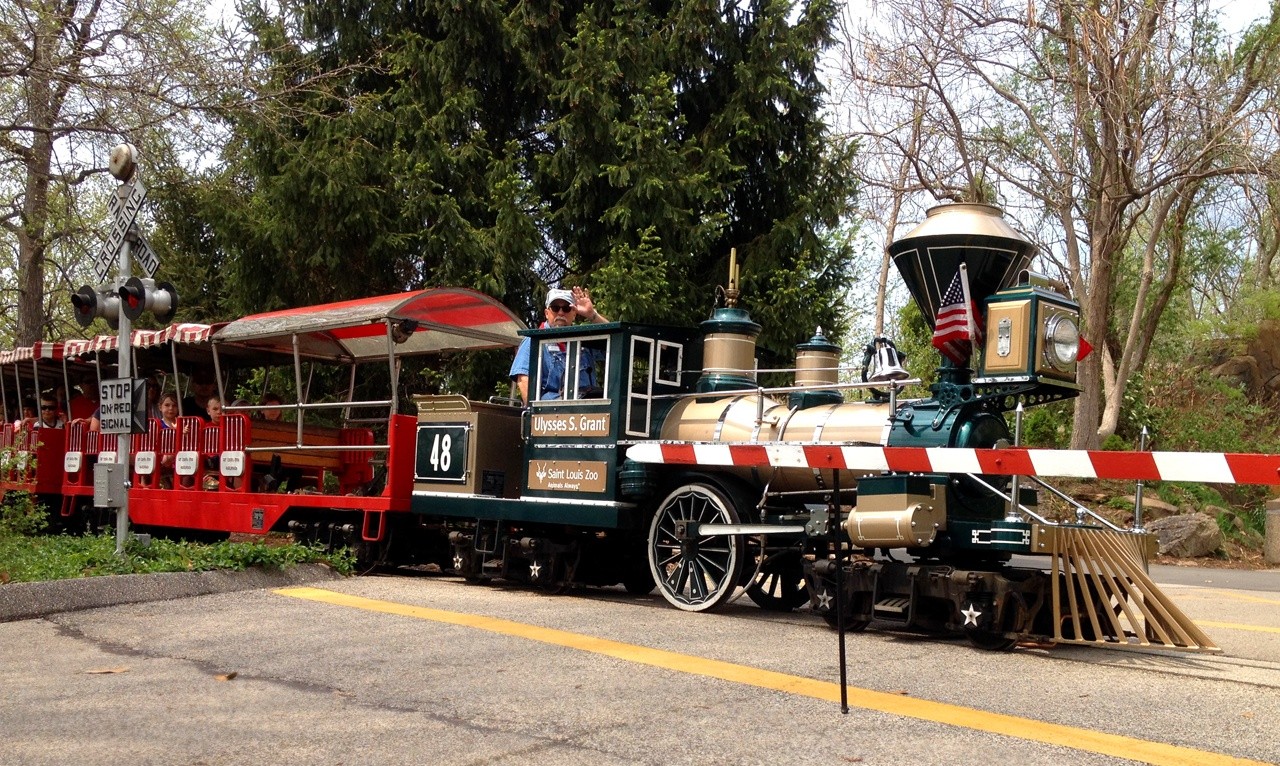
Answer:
<box><xmin>193</xmin><ymin>379</ymin><xmax>215</xmax><ymax>385</ymax></box>
<box><xmin>41</xmin><ymin>405</ymin><xmax>57</xmax><ymax>410</ymax></box>
<box><xmin>549</xmin><ymin>305</ymin><xmax>572</xmax><ymax>313</ymax></box>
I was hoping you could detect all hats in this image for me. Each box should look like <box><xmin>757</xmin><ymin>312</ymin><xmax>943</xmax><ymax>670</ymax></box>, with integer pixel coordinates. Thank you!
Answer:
<box><xmin>545</xmin><ymin>288</ymin><xmax>577</xmax><ymax>309</ymax></box>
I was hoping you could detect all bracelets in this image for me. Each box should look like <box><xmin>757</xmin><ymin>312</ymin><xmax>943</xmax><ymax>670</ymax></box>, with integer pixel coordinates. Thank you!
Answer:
<box><xmin>588</xmin><ymin>309</ymin><xmax>597</xmax><ymax>321</ymax></box>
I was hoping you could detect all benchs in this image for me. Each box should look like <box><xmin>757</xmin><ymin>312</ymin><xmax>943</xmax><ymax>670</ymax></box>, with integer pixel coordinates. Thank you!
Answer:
<box><xmin>0</xmin><ymin>403</ymin><xmax>380</xmax><ymax>494</ymax></box>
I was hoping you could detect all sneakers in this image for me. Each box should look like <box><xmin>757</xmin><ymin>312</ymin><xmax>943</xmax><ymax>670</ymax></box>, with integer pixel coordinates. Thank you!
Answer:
<box><xmin>203</xmin><ymin>475</ymin><xmax>219</xmax><ymax>490</ymax></box>
<box><xmin>160</xmin><ymin>478</ymin><xmax>171</xmax><ymax>488</ymax></box>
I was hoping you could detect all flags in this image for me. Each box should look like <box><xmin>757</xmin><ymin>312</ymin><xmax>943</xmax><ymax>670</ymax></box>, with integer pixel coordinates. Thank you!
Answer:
<box><xmin>932</xmin><ymin>270</ymin><xmax>984</xmax><ymax>371</ymax></box>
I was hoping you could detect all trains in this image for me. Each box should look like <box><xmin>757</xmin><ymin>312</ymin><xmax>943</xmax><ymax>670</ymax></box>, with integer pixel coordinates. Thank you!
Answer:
<box><xmin>1</xmin><ymin>204</ymin><xmax>1223</xmax><ymax>654</ymax></box>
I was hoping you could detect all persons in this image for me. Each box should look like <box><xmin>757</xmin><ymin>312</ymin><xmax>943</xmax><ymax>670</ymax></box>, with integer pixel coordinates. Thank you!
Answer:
<box><xmin>1</xmin><ymin>372</ymin><xmax>300</xmax><ymax>494</ymax></box>
<box><xmin>509</xmin><ymin>285</ymin><xmax>610</xmax><ymax>410</ymax></box>
